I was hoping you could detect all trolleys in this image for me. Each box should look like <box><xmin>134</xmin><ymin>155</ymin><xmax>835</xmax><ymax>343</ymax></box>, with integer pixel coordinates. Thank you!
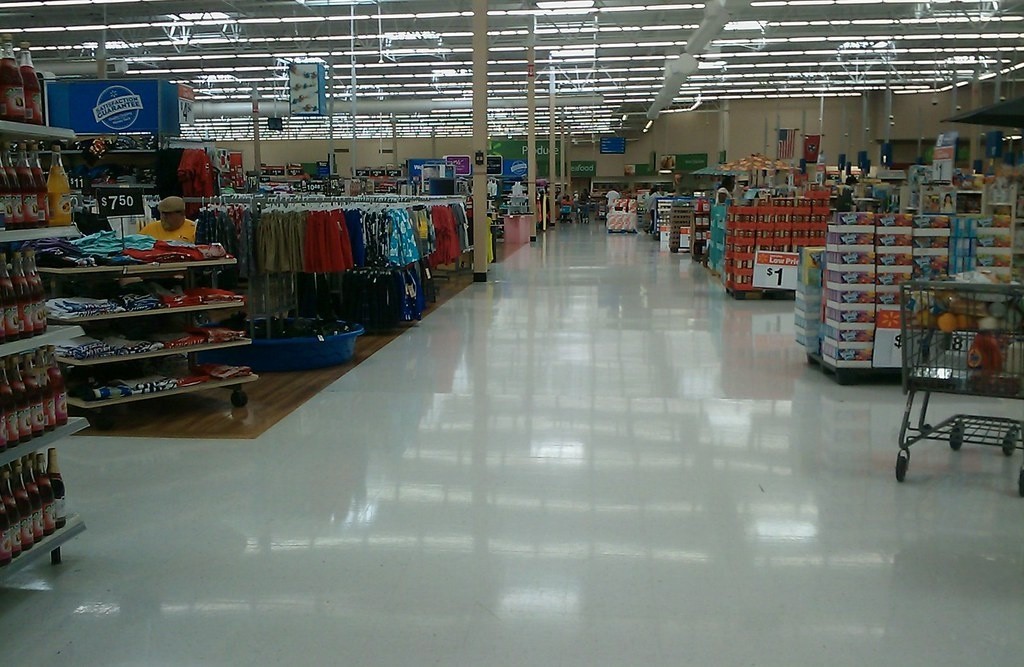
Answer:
<box><xmin>895</xmin><ymin>280</ymin><xmax>1024</xmax><ymax>497</ymax></box>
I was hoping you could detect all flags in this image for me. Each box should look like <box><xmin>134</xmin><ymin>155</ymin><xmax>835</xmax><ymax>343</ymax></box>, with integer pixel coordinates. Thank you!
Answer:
<box><xmin>778</xmin><ymin>129</ymin><xmax>794</xmax><ymax>159</ymax></box>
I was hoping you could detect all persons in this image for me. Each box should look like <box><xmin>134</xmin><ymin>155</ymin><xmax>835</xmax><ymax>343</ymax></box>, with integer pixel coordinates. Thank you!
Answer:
<box><xmin>139</xmin><ymin>196</ymin><xmax>198</xmax><ymax>246</ymax></box>
<box><xmin>942</xmin><ymin>194</ymin><xmax>952</xmax><ymax>212</ymax></box>
<box><xmin>562</xmin><ymin>195</ymin><xmax>572</xmax><ymax>205</ymax></box>
<box><xmin>580</xmin><ymin>189</ymin><xmax>590</xmax><ymax>224</ymax></box>
<box><xmin>644</xmin><ymin>186</ymin><xmax>664</xmax><ymax>234</ymax></box>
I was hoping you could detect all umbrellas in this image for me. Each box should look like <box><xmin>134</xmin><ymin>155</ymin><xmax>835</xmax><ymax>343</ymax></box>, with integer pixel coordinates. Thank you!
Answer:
<box><xmin>690</xmin><ymin>162</ymin><xmax>747</xmax><ymax>189</ymax></box>
<box><xmin>721</xmin><ymin>153</ymin><xmax>802</xmax><ymax>187</ymax></box>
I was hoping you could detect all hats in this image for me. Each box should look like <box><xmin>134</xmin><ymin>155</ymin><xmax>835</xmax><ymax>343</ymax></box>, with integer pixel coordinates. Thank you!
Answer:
<box><xmin>157</xmin><ymin>196</ymin><xmax>185</xmax><ymax>212</ymax></box>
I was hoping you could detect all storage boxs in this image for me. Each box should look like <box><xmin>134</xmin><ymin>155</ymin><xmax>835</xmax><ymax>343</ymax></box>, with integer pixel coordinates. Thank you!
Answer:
<box><xmin>792</xmin><ymin>212</ymin><xmax>1011</xmax><ymax>363</ymax></box>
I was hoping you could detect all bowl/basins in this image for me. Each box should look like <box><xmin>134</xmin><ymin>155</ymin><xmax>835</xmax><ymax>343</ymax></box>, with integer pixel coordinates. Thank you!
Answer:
<box><xmin>197</xmin><ymin>318</ymin><xmax>364</xmax><ymax>372</ymax></box>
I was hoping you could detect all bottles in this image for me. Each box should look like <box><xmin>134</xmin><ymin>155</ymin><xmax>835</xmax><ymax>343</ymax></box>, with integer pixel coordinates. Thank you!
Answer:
<box><xmin>0</xmin><ymin>250</ymin><xmax>47</xmax><ymax>345</ymax></box>
<box><xmin>19</xmin><ymin>41</ymin><xmax>42</xmax><ymax>124</ymax></box>
<box><xmin>0</xmin><ymin>346</ymin><xmax>67</xmax><ymax>566</ymax></box>
<box><xmin>0</xmin><ymin>34</ymin><xmax>26</xmax><ymax>123</ymax></box>
<box><xmin>0</xmin><ymin>141</ymin><xmax>71</xmax><ymax>231</ymax></box>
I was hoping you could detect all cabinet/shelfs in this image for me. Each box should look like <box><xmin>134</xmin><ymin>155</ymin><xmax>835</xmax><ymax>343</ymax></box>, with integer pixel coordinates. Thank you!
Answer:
<box><xmin>0</xmin><ymin>119</ymin><xmax>87</xmax><ymax>589</ymax></box>
<box><xmin>654</xmin><ymin>163</ymin><xmax>838</xmax><ymax>300</ymax></box>
<box><xmin>43</xmin><ymin>78</ymin><xmax>195</xmax><ymax>194</ymax></box>
<box><xmin>33</xmin><ymin>252</ymin><xmax>258</xmax><ymax>431</ymax></box>
<box><xmin>485</xmin><ymin>175</ymin><xmax>568</xmax><ymax>263</ymax></box>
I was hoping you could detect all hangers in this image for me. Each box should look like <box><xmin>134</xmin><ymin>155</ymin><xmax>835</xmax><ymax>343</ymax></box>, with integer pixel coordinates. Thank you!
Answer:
<box><xmin>68</xmin><ymin>194</ymin><xmax>161</xmax><ymax>203</ymax></box>
<box><xmin>202</xmin><ymin>191</ymin><xmax>466</xmax><ymax>214</ymax></box>
